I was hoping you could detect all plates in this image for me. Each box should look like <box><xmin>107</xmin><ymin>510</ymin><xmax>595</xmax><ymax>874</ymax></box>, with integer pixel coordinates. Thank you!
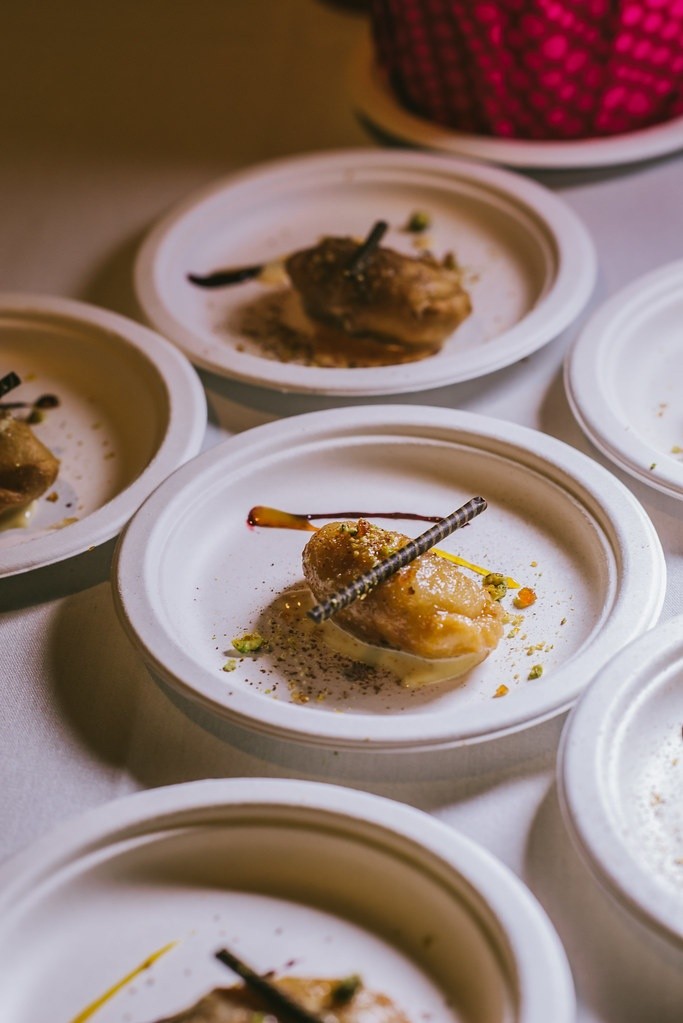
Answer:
<box><xmin>130</xmin><ymin>146</ymin><xmax>600</xmax><ymax>398</ymax></box>
<box><xmin>553</xmin><ymin>613</ymin><xmax>683</xmax><ymax>953</ymax></box>
<box><xmin>109</xmin><ymin>404</ymin><xmax>670</xmax><ymax>757</ymax></box>
<box><xmin>0</xmin><ymin>287</ymin><xmax>208</xmax><ymax>580</ymax></box>
<box><xmin>561</xmin><ymin>260</ymin><xmax>683</xmax><ymax>503</ymax></box>
<box><xmin>0</xmin><ymin>776</ymin><xmax>578</xmax><ymax>1023</ymax></box>
<box><xmin>347</xmin><ymin>37</ymin><xmax>683</xmax><ymax>168</ymax></box>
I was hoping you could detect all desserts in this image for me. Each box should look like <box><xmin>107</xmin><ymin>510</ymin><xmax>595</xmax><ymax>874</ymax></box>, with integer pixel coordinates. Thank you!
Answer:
<box><xmin>147</xmin><ymin>947</ymin><xmax>412</xmax><ymax>1022</ymax></box>
<box><xmin>281</xmin><ymin>219</ymin><xmax>473</xmax><ymax>356</ymax></box>
<box><xmin>301</xmin><ymin>495</ymin><xmax>509</xmax><ymax>690</ymax></box>
<box><xmin>0</xmin><ymin>405</ymin><xmax>60</xmax><ymax>528</ymax></box>
